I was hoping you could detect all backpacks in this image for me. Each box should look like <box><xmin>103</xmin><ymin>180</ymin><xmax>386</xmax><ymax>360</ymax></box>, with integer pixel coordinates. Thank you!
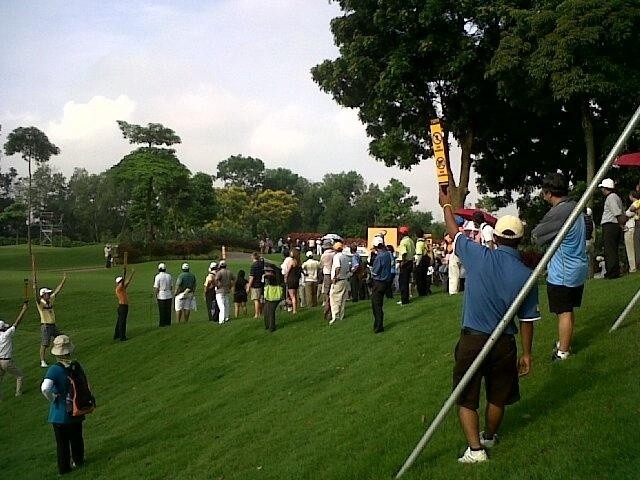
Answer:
<box><xmin>57</xmin><ymin>361</ymin><xmax>97</xmax><ymax>416</ymax></box>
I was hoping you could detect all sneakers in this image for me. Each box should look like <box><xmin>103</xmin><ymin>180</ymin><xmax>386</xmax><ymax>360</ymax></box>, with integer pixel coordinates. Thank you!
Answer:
<box><xmin>552</xmin><ymin>341</ymin><xmax>573</xmax><ymax>352</ymax></box>
<box><xmin>73</xmin><ymin>456</ymin><xmax>86</xmax><ymax>467</ymax></box>
<box><xmin>458</xmin><ymin>446</ymin><xmax>487</xmax><ymax>464</ymax></box>
<box><xmin>40</xmin><ymin>360</ymin><xmax>50</xmax><ymax>368</ymax></box>
<box><xmin>551</xmin><ymin>351</ymin><xmax>571</xmax><ymax>361</ymax></box>
<box><xmin>14</xmin><ymin>391</ymin><xmax>26</xmax><ymax>397</ymax></box>
<box><xmin>479</xmin><ymin>431</ymin><xmax>498</xmax><ymax>449</ymax></box>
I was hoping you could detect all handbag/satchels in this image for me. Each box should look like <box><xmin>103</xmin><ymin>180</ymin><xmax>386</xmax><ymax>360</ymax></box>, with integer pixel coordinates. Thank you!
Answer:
<box><xmin>263</xmin><ymin>284</ymin><xmax>284</xmax><ymax>302</ymax></box>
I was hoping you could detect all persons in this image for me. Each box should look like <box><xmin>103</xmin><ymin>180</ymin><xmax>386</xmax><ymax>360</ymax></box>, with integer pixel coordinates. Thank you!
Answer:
<box><xmin>112</xmin><ymin>267</ymin><xmax>135</xmax><ymax>342</ymax></box>
<box><xmin>32</xmin><ymin>271</ymin><xmax>68</xmax><ymax>368</ymax></box>
<box><xmin>583</xmin><ymin>184</ymin><xmax>640</xmax><ymax>282</ymax></box>
<box><xmin>153</xmin><ymin>262</ymin><xmax>175</xmax><ymax>327</ymax></box>
<box><xmin>40</xmin><ymin>335</ymin><xmax>93</xmax><ymax>477</ymax></box>
<box><xmin>437</xmin><ymin>181</ymin><xmax>543</xmax><ymax>465</ymax></box>
<box><xmin>0</xmin><ymin>299</ymin><xmax>28</xmax><ymax>399</ymax></box>
<box><xmin>173</xmin><ymin>262</ymin><xmax>197</xmax><ymax>326</ymax></box>
<box><xmin>530</xmin><ymin>170</ymin><xmax>590</xmax><ymax>365</ymax></box>
<box><xmin>104</xmin><ymin>242</ymin><xmax>113</xmax><ymax>268</ymax></box>
<box><xmin>202</xmin><ymin>208</ymin><xmax>499</xmax><ymax>336</ymax></box>
<box><xmin>598</xmin><ymin>178</ymin><xmax>627</xmax><ymax>280</ymax></box>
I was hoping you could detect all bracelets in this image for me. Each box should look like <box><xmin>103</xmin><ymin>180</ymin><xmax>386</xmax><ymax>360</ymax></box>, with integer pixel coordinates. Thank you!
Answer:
<box><xmin>442</xmin><ymin>203</ymin><xmax>453</xmax><ymax>212</ymax></box>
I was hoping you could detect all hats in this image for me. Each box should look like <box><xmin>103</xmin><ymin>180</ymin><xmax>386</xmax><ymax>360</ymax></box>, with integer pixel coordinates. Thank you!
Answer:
<box><xmin>262</xmin><ymin>263</ymin><xmax>273</xmax><ymax>270</ymax></box>
<box><xmin>305</xmin><ymin>250</ymin><xmax>314</xmax><ymax>258</ymax></box>
<box><xmin>0</xmin><ymin>320</ymin><xmax>11</xmax><ymax>332</ymax></box>
<box><xmin>50</xmin><ymin>334</ymin><xmax>78</xmax><ymax>356</ymax></box>
<box><xmin>597</xmin><ymin>177</ymin><xmax>618</xmax><ymax>190</ymax></box>
<box><xmin>333</xmin><ymin>242</ymin><xmax>344</xmax><ymax>251</ymax></box>
<box><xmin>182</xmin><ymin>262</ymin><xmax>189</xmax><ymax>269</ymax></box>
<box><xmin>321</xmin><ymin>240</ymin><xmax>333</xmax><ymax>249</ymax></box>
<box><xmin>38</xmin><ymin>288</ymin><xmax>54</xmax><ymax>297</ymax></box>
<box><xmin>372</xmin><ymin>237</ymin><xmax>384</xmax><ymax>248</ymax></box>
<box><xmin>399</xmin><ymin>225</ymin><xmax>410</xmax><ymax>235</ymax></box>
<box><xmin>157</xmin><ymin>263</ymin><xmax>167</xmax><ymax>270</ymax></box>
<box><xmin>208</xmin><ymin>260</ymin><xmax>227</xmax><ymax>272</ymax></box>
<box><xmin>116</xmin><ymin>277</ymin><xmax>124</xmax><ymax>283</ymax></box>
<box><xmin>494</xmin><ymin>216</ymin><xmax>528</xmax><ymax>239</ymax></box>
